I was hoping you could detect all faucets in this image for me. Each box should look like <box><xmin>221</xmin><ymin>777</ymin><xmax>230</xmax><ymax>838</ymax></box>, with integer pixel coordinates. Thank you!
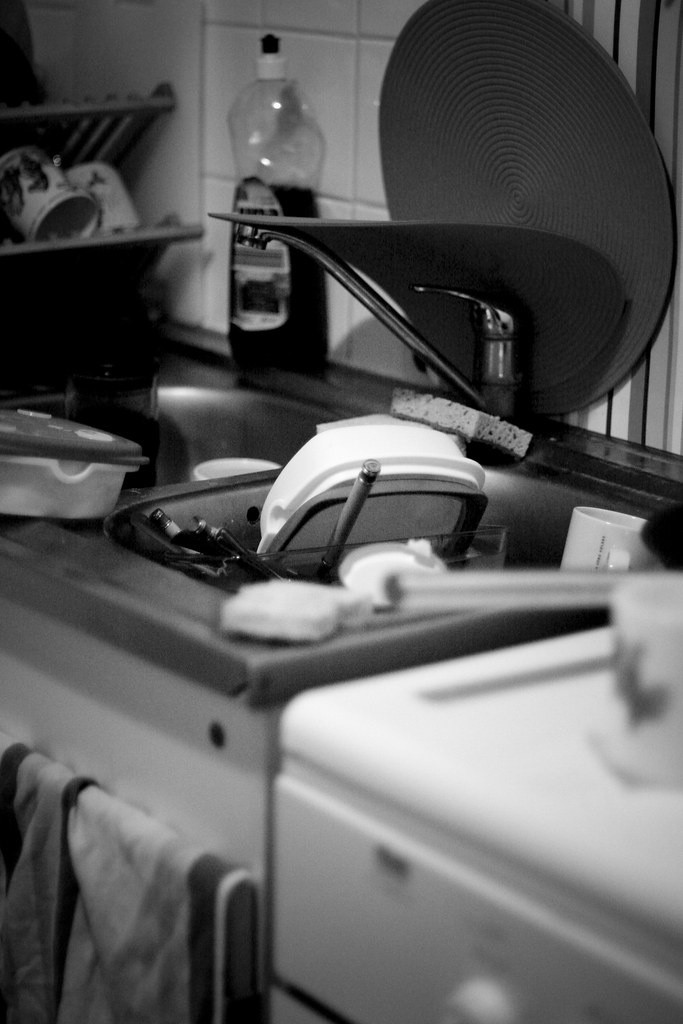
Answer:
<box><xmin>228</xmin><ymin>220</ymin><xmax>528</xmax><ymax>421</ymax></box>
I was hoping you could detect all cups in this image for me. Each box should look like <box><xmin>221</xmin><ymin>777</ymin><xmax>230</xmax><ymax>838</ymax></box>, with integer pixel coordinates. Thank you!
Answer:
<box><xmin>193</xmin><ymin>457</ymin><xmax>283</xmax><ymax>480</ymax></box>
<box><xmin>596</xmin><ymin>570</ymin><xmax>683</xmax><ymax>792</ymax></box>
<box><xmin>559</xmin><ymin>505</ymin><xmax>662</xmax><ymax>576</ymax></box>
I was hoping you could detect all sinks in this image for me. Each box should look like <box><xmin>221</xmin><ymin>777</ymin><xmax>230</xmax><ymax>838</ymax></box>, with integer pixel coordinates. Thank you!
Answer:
<box><xmin>0</xmin><ymin>360</ymin><xmax>357</xmax><ymax>530</ymax></box>
<box><xmin>98</xmin><ymin>444</ymin><xmax>683</xmax><ymax>610</ymax></box>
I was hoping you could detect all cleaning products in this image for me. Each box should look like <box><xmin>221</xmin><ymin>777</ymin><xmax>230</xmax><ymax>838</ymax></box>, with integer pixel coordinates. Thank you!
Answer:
<box><xmin>224</xmin><ymin>24</ymin><xmax>334</xmax><ymax>372</ymax></box>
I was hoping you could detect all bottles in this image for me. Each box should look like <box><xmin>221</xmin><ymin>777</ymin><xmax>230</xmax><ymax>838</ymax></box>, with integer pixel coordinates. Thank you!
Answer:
<box><xmin>226</xmin><ymin>34</ymin><xmax>329</xmax><ymax>376</ymax></box>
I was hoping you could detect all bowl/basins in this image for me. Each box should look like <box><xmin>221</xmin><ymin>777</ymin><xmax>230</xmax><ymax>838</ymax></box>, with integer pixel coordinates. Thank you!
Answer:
<box><xmin>0</xmin><ymin>406</ymin><xmax>151</xmax><ymax>519</ymax></box>
